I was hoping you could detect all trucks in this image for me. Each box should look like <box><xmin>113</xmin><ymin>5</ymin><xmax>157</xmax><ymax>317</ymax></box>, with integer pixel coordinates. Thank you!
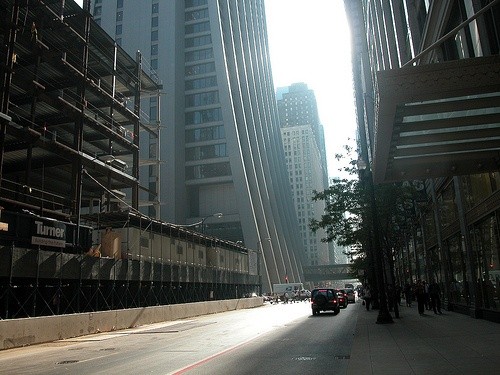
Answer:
<box><xmin>272</xmin><ymin>282</ymin><xmax>311</xmax><ymax>301</ymax></box>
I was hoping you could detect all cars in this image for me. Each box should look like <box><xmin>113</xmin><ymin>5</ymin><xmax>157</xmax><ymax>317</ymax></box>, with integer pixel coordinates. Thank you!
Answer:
<box><xmin>344</xmin><ymin>283</ymin><xmax>365</xmax><ymax>302</ymax></box>
<box><xmin>329</xmin><ymin>289</ymin><xmax>349</xmax><ymax>308</ymax></box>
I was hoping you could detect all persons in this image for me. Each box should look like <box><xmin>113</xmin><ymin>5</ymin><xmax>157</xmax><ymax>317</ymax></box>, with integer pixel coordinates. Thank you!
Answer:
<box><xmin>395</xmin><ymin>279</ymin><xmax>441</xmax><ymax>315</ymax></box>
<box><xmin>283</xmin><ymin>287</ymin><xmax>309</xmax><ymax>304</ymax></box>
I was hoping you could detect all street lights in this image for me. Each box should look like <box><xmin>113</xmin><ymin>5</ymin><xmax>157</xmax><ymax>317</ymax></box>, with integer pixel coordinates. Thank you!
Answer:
<box><xmin>201</xmin><ymin>212</ymin><xmax>224</xmax><ymax>234</ymax></box>
<box><xmin>75</xmin><ymin>155</ymin><xmax>127</xmax><ymax>254</ymax></box>
<box><xmin>256</xmin><ymin>238</ymin><xmax>272</xmax><ymax>275</ymax></box>
<box><xmin>356</xmin><ymin>158</ymin><xmax>393</xmax><ymax>326</ymax></box>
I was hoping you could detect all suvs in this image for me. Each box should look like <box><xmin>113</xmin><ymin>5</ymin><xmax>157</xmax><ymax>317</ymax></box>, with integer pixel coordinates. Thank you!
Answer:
<box><xmin>311</xmin><ymin>288</ymin><xmax>342</xmax><ymax>316</ymax></box>
